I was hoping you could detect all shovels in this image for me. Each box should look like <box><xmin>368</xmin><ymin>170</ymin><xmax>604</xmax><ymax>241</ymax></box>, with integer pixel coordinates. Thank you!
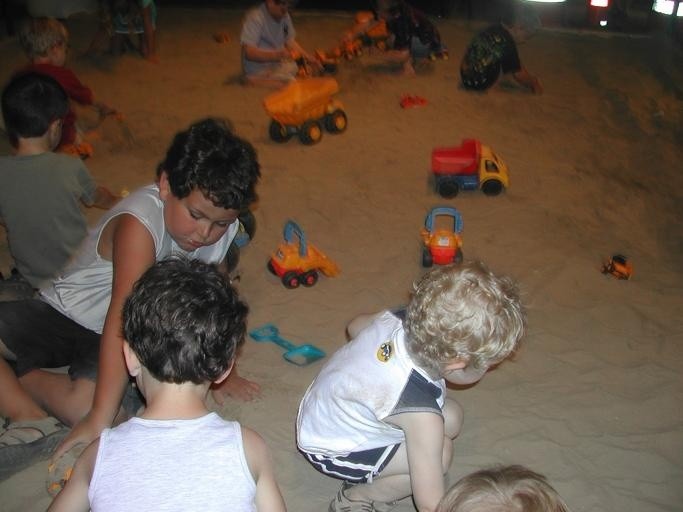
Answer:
<box><xmin>249</xmin><ymin>324</ymin><xmax>326</xmax><ymax>366</ymax></box>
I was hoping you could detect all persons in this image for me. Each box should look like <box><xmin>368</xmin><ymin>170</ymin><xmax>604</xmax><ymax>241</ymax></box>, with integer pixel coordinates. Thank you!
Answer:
<box><xmin>238</xmin><ymin>0</ymin><xmax>309</xmax><ymax>84</ymax></box>
<box><xmin>460</xmin><ymin>3</ymin><xmax>544</xmax><ymax>94</ymax></box>
<box><xmin>47</xmin><ymin>253</ymin><xmax>288</xmax><ymax>512</ymax></box>
<box><xmin>15</xmin><ymin>16</ymin><xmax>117</xmax><ymax>147</ymax></box>
<box><xmin>0</xmin><ymin>72</ymin><xmax>124</xmax><ymax>290</ymax></box>
<box><xmin>436</xmin><ymin>465</ymin><xmax>569</xmax><ymax>512</ymax></box>
<box><xmin>295</xmin><ymin>258</ymin><xmax>527</xmax><ymax>512</ymax></box>
<box><xmin>0</xmin><ymin>116</ymin><xmax>263</xmax><ymax>466</ymax></box>
<box><xmin>88</xmin><ymin>0</ymin><xmax>158</xmax><ymax>57</ymax></box>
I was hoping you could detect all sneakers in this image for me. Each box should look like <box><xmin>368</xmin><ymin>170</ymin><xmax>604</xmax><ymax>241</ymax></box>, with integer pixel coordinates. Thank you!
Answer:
<box><xmin>0</xmin><ymin>417</ymin><xmax>69</xmax><ymax>480</ymax></box>
<box><xmin>328</xmin><ymin>482</ymin><xmax>376</xmax><ymax>512</ymax></box>
